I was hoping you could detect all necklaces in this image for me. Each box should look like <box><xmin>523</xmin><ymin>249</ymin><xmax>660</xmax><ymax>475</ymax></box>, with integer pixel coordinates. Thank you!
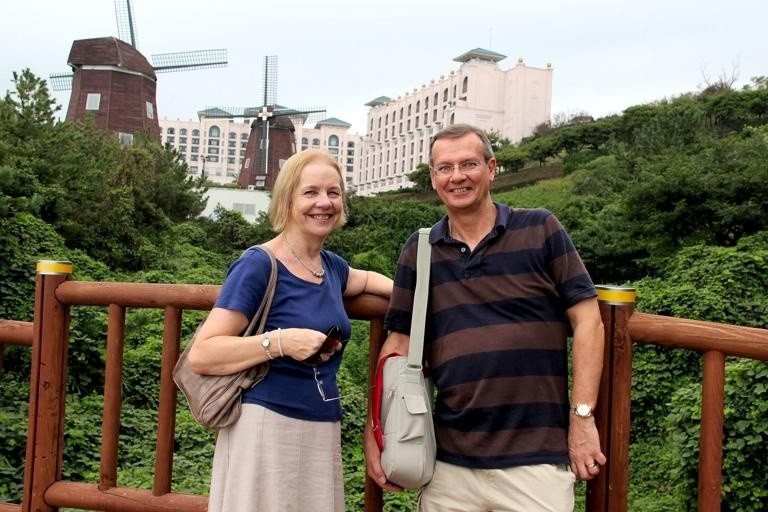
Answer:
<box><xmin>282</xmin><ymin>229</ymin><xmax>326</xmax><ymax>277</ymax></box>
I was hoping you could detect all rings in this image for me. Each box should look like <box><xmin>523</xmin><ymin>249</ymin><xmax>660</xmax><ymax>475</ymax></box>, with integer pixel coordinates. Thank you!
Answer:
<box><xmin>586</xmin><ymin>462</ymin><xmax>596</xmax><ymax>469</ymax></box>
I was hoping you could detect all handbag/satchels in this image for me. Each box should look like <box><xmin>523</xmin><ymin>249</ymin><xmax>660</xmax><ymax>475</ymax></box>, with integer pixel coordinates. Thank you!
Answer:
<box><xmin>380</xmin><ymin>356</ymin><xmax>437</xmax><ymax>489</ymax></box>
<box><xmin>173</xmin><ymin>315</ymin><xmax>268</xmax><ymax>428</ymax></box>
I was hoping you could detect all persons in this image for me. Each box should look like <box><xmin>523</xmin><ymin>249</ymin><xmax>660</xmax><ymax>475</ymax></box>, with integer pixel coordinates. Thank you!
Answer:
<box><xmin>361</xmin><ymin>122</ymin><xmax>608</xmax><ymax>512</ymax></box>
<box><xmin>185</xmin><ymin>146</ymin><xmax>394</xmax><ymax>512</ymax></box>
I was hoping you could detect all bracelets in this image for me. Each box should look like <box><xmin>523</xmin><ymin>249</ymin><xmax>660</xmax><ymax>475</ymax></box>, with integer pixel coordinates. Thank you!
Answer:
<box><xmin>275</xmin><ymin>325</ymin><xmax>284</xmax><ymax>358</ymax></box>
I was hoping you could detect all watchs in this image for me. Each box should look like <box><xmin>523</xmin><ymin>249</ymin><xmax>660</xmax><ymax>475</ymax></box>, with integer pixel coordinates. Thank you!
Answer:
<box><xmin>567</xmin><ymin>403</ymin><xmax>595</xmax><ymax>418</ymax></box>
<box><xmin>261</xmin><ymin>329</ymin><xmax>275</xmax><ymax>362</ymax></box>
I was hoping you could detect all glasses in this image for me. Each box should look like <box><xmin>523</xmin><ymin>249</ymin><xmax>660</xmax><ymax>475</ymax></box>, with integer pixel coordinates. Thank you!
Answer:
<box><xmin>433</xmin><ymin>161</ymin><xmax>480</xmax><ymax>178</ymax></box>
<box><xmin>299</xmin><ymin>325</ymin><xmax>341</xmax><ymax>367</ymax></box>
